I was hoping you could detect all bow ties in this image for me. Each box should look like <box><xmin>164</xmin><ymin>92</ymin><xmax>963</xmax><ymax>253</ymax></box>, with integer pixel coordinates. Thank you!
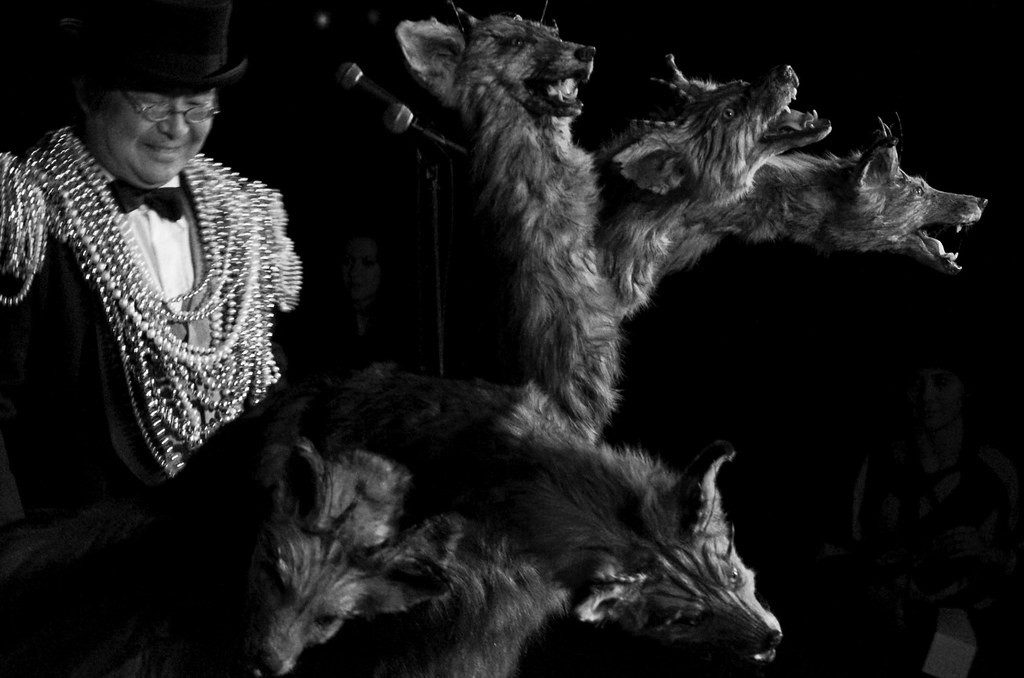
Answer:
<box><xmin>111</xmin><ymin>178</ymin><xmax>183</xmax><ymax>221</ymax></box>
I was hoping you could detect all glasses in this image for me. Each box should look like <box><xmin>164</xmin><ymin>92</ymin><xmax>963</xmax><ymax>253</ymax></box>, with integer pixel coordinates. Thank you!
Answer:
<box><xmin>121</xmin><ymin>90</ymin><xmax>219</xmax><ymax>124</ymax></box>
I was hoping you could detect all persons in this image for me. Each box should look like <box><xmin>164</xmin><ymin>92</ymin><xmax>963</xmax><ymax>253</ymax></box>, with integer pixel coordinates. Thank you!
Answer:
<box><xmin>0</xmin><ymin>0</ymin><xmax>308</xmax><ymax>525</ymax></box>
<box><xmin>320</xmin><ymin>229</ymin><xmax>409</xmax><ymax>366</ymax></box>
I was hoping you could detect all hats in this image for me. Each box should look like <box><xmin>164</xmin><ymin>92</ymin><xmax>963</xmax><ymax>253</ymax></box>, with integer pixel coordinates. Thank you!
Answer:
<box><xmin>60</xmin><ymin>0</ymin><xmax>249</xmax><ymax>92</ymax></box>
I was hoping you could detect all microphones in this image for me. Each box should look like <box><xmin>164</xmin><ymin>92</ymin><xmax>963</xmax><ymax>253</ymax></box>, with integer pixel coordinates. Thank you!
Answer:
<box><xmin>337</xmin><ymin>61</ymin><xmax>398</xmax><ymax>108</ymax></box>
<box><xmin>384</xmin><ymin>102</ymin><xmax>467</xmax><ymax>160</ymax></box>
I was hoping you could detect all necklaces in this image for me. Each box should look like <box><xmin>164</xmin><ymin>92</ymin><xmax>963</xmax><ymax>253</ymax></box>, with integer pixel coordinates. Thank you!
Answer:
<box><xmin>0</xmin><ymin>123</ymin><xmax>303</xmax><ymax>477</ymax></box>
